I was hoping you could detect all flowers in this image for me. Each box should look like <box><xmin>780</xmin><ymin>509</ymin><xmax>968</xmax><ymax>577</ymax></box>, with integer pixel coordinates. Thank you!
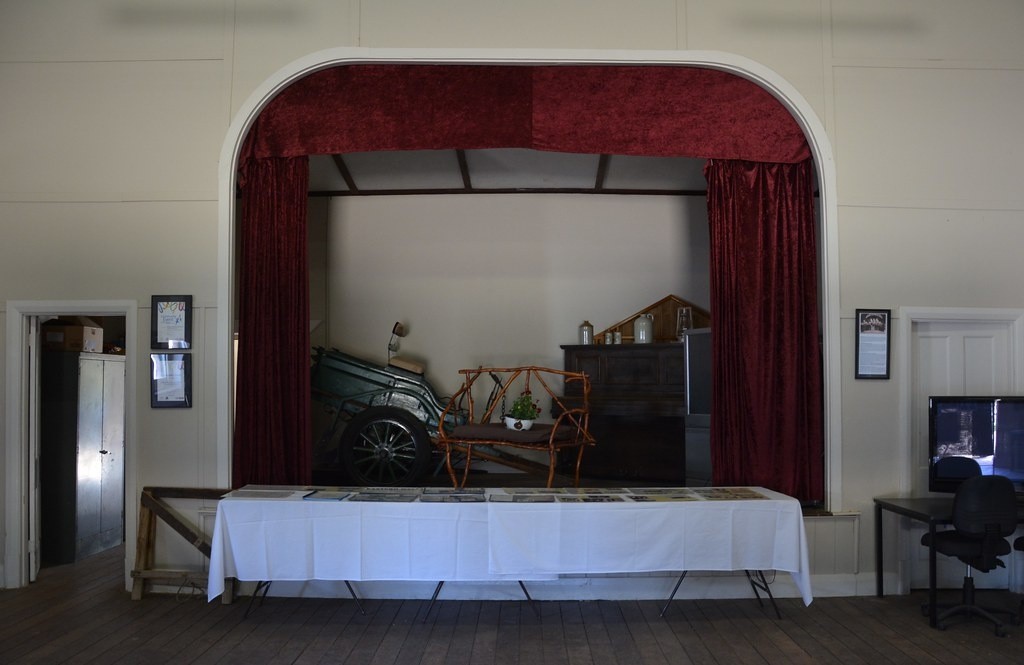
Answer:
<box><xmin>510</xmin><ymin>390</ymin><xmax>543</xmax><ymax>419</ymax></box>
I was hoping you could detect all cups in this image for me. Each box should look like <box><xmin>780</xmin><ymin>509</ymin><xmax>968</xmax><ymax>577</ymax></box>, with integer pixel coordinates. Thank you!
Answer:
<box><xmin>614</xmin><ymin>332</ymin><xmax>621</xmax><ymax>344</ymax></box>
<box><xmin>605</xmin><ymin>332</ymin><xmax>612</xmax><ymax>344</ymax></box>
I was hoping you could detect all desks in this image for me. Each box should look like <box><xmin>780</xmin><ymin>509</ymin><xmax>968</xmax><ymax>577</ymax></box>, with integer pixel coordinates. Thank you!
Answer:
<box><xmin>871</xmin><ymin>491</ymin><xmax>1023</xmax><ymax>629</ymax></box>
<box><xmin>207</xmin><ymin>482</ymin><xmax>806</xmax><ymax>623</ymax></box>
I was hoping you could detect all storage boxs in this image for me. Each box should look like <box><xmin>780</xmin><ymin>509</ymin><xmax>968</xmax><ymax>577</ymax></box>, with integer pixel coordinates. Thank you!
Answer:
<box><xmin>40</xmin><ymin>315</ymin><xmax>104</xmax><ymax>351</ymax></box>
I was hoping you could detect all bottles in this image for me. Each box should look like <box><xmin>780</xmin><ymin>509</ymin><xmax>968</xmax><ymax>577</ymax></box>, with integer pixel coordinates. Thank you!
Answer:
<box><xmin>633</xmin><ymin>313</ymin><xmax>653</xmax><ymax>344</ymax></box>
<box><xmin>578</xmin><ymin>321</ymin><xmax>594</xmax><ymax>345</ymax></box>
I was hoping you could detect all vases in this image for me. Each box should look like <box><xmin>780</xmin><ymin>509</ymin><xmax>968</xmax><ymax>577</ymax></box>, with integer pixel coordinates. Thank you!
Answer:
<box><xmin>505</xmin><ymin>413</ymin><xmax>536</xmax><ymax>431</ymax></box>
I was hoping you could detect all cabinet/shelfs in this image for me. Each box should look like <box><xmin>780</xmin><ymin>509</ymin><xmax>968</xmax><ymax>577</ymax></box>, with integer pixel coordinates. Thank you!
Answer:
<box><xmin>41</xmin><ymin>348</ymin><xmax>127</xmax><ymax>561</ymax></box>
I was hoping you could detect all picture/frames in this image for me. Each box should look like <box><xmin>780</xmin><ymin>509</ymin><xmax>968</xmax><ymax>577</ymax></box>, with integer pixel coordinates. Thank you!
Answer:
<box><xmin>151</xmin><ymin>352</ymin><xmax>192</xmax><ymax>408</ymax></box>
<box><xmin>853</xmin><ymin>307</ymin><xmax>892</xmax><ymax>380</ymax></box>
<box><xmin>149</xmin><ymin>295</ymin><xmax>195</xmax><ymax>350</ymax></box>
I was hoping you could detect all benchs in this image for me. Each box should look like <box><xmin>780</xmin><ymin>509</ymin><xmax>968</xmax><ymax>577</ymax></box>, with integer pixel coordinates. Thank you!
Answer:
<box><xmin>437</xmin><ymin>366</ymin><xmax>596</xmax><ymax>490</ymax></box>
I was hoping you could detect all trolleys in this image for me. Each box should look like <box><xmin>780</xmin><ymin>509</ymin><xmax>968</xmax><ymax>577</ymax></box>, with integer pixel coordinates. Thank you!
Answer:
<box><xmin>307</xmin><ymin>346</ymin><xmax>590</xmax><ymax>489</ymax></box>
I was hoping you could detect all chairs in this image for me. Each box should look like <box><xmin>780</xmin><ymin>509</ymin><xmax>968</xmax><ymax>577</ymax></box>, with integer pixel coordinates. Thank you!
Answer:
<box><xmin>917</xmin><ymin>473</ymin><xmax>1019</xmax><ymax>640</ymax></box>
